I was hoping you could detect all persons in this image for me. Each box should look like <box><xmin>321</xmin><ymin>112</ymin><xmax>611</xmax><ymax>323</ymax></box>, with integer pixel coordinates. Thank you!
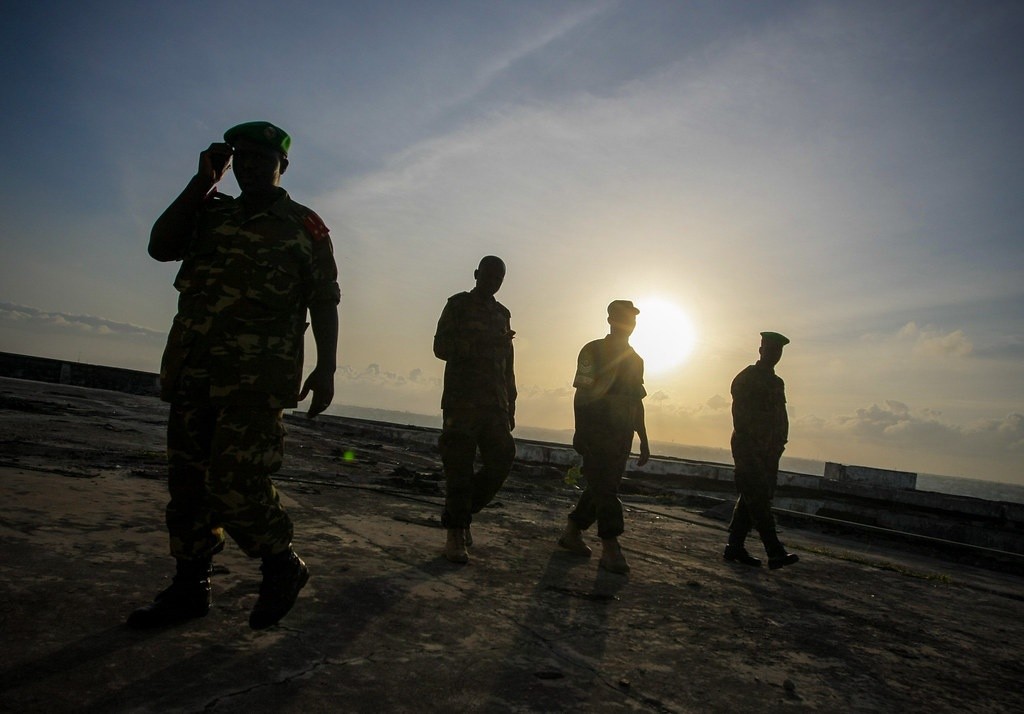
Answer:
<box><xmin>129</xmin><ymin>121</ymin><xmax>342</xmax><ymax>631</ymax></box>
<box><xmin>559</xmin><ymin>301</ymin><xmax>651</xmax><ymax>574</ymax></box>
<box><xmin>724</xmin><ymin>332</ymin><xmax>799</xmax><ymax>569</ymax></box>
<box><xmin>433</xmin><ymin>256</ymin><xmax>517</xmax><ymax>562</ymax></box>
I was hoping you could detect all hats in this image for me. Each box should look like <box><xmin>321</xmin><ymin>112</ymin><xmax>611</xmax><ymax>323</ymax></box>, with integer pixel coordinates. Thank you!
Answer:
<box><xmin>608</xmin><ymin>300</ymin><xmax>640</xmax><ymax>317</ymax></box>
<box><xmin>224</xmin><ymin>121</ymin><xmax>290</xmax><ymax>158</ymax></box>
<box><xmin>761</xmin><ymin>333</ymin><xmax>789</xmax><ymax>349</ymax></box>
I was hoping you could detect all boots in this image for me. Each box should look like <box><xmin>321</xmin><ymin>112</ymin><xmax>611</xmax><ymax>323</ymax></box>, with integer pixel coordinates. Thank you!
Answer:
<box><xmin>559</xmin><ymin>517</ymin><xmax>593</xmax><ymax>556</ymax></box>
<box><xmin>600</xmin><ymin>537</ymin><xmax>630</xmax><ymax>573</ymax></box>
<box><xmin>128</xmin><ymin>559</ymin><xmax>213</xmax><ymax>629</ymax></box>
<box><xmin>443</xmin><ymin>528</ymin><xmax>470</xmax><ymax>562</ymax></box>
<box><xmin>466</xmin><ymin>529</ymin><xmax>473</xmax><ymax>544</ymax></box>
<box><xmin>767</xmin><ymin>541</ymin><xmax>800</xmax><ymax>568</ymax></box>
<box><xmin>247</xmin><ymin>542</ymin><xmax>311</xmax><ymax>628</ymax></box>
<box><xmin>725</xmin><ymin>532</ymin><xmax>761</xmax><ymax>567</ymax></box>
